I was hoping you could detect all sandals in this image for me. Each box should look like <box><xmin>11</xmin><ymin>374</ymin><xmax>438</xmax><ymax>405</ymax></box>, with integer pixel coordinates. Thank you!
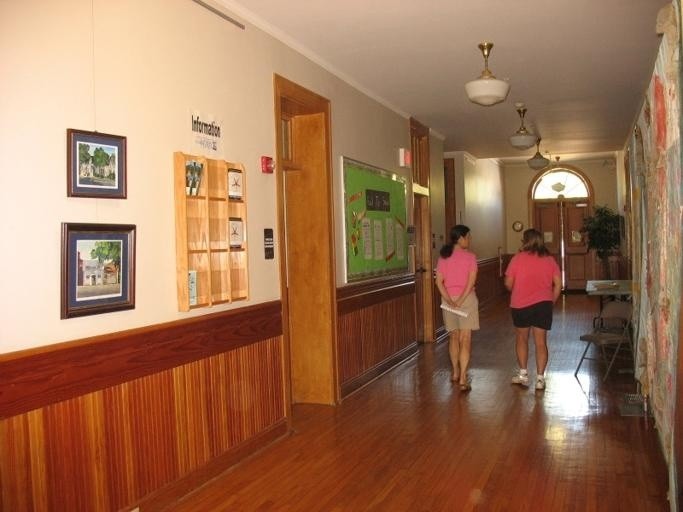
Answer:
<box><xmin>451</xmin><ymin>372</ymin><xmax>470</xmax><ymax>391</ymax></box>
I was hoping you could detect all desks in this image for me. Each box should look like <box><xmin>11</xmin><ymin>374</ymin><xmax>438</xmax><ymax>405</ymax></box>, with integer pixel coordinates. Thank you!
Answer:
<box><xmin>586</xmin><ymin>280</ymin><xmax>633</xmax><ymax>328</ymax></box>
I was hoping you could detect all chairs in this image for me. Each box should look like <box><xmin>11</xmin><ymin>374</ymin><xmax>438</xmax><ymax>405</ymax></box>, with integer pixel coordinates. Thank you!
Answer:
<box><xmin>574</xmin><ymin>301</ymin><xmax>633</xmax><ymax>381</ymax></box>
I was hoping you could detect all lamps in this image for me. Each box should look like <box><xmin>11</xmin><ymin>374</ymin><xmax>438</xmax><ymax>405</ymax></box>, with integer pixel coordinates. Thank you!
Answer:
<box><xmin>464</xmin><ymin>43</ymin><xmax>564</xmax><ymax>191</ymax></box>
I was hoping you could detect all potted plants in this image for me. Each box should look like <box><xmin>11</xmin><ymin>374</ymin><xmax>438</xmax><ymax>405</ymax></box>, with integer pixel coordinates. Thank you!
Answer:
<box><xmin>573</xmin><ymin>204</ymin><xmax>625</xmax><ymax>280</ymax></box>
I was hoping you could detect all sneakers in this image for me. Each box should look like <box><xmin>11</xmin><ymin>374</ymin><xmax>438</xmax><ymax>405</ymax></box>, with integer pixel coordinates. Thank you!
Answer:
<box><xmin>534</xmin><ymin>377</ymin><xmax>545</xmax><ymax>389</ymax></box>
<box><xmin>512</xmin><ymin>374</ymin><xmax>529</xmax><ymax>385</ymax></box>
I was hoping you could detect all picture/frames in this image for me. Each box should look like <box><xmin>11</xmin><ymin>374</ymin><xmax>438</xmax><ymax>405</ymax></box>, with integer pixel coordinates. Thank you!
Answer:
<box><xmin>60</xmin><ymin>223</ymin><xmax>136</xmax><ymax>320</ymax></box>
<box><xmin>67</xmin><ymin>128</ymin><xmax>127</xmax><ymax>199</ymax></box>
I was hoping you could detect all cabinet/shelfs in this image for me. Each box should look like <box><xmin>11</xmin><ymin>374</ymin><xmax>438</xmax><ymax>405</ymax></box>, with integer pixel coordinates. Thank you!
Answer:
<box><xmin>174</xmin><ymin>152</ymin><xmax>249</xmax><ymax>312</ymax></box>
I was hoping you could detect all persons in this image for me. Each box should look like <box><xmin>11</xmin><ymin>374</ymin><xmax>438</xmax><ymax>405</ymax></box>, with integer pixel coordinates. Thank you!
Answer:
<box><xmin>434</xmin><ymin>224</ymin><xmax>480</xmax><ymax>392</ymax></box>
<box><xmin>503</xmin><ymin>228</ymin><xmax>562</xmax><ymax>390</ymax></box>
